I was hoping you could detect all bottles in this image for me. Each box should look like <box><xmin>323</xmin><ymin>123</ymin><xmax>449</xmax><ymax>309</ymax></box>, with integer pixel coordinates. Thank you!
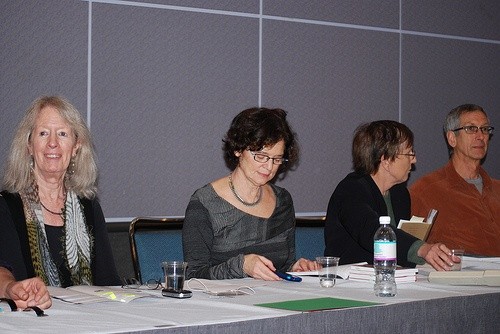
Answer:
<box><xmin>373</xmin><ymin>216</ymin><xmax>397</xmax><ymax>298</ymax></box>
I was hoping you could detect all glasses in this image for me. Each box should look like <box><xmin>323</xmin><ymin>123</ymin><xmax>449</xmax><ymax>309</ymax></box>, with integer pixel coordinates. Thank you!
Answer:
<box><xmin>248</xmin><ymin>150</ymin><xmax>288</xmax><ymax>165</ymax></box>
<box><xmin>398</xmin><ymin>151</ymin><xmax>416</xmax><ymax>160</ymax></box>
<box><xmin>453</xmin><ymin>126</ymin><xmax>495</xmax><ymax>134</ymax></box>
<box><xmin>121</xmin><ymin>277</ymin><xmax>164</xmax><ymax>290</ymax></box>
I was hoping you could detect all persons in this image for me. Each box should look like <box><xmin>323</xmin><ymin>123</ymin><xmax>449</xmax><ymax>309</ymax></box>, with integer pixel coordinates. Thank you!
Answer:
<box><xmin>182</xmin><ymin>107</ymin><xmax>322</xmax><ymax>281</ymax></box>
<box><xmin>0</xmin><ymin>94</ymin><xmax>119</xmax><ymax>288</ymax></box>
<box><xmin>324</xmin><ymin>120</ymin><xmax>461</xmax><ymax>272</ymax></box>
<box><xmin>408</xmin><ymin>103</ymin><xmax>500</xmax><ymax>257</ymax></box>
<box><xmin>0</xmin><ymin>266</ymin><xmax>52</xmax><ymax>311</ymax></box>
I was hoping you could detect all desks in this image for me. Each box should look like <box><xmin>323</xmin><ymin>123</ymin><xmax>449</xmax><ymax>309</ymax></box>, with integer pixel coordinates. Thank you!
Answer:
<box><xmin>0</xmin><ymin>255</ymin><xmax>500</xmax><ymax>334</ymax></box>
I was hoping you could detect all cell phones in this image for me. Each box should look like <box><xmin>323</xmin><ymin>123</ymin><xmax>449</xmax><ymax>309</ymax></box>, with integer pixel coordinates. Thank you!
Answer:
<box><xmin>161</xmin><ymin>288</ymin><xmax>193</xmax><ymax>299</ymax></box>
<box><xmin>272</xmin><ymin>269</ymin><xmax>303</xmax><ymax>283</ymax></box>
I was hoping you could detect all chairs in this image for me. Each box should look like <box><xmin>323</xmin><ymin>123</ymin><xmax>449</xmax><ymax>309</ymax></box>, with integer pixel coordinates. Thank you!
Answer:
<box><xmin>294</xmin><ymin>217</ymin><xmax>326</xmax><ymax>267</ymax></box>
<box><xmin>127</xmin><ymin>216</ymin><xmax>184</xmax><ymax>284</ymax></box>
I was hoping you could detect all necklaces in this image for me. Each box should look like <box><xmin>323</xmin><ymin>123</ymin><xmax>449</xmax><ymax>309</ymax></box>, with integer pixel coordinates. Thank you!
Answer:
<box><xmin>40</xmin><ymin>200</ymin><xmax>63</xmax><ymax>214</ymax></box>
<box><xmin>228</xmin><ymin>173</ymin><xmax>262</xmax><ymax>205</ymax></box>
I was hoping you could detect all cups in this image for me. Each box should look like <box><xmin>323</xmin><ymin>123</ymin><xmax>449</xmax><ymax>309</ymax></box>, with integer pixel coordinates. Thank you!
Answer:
<box><xmin>442</xmin><ymin>248</ymin><xmax>464</xmax><ymax>271</ymax></box>
<box><xmin>161</xmin><ymin>261</ymin><xmax>188</xmax><ymax>290</ymax></box>
<box><xmin>315</xmin><ymin>256</ymin><xmax>340</xmax><ymax>289</ymax></box>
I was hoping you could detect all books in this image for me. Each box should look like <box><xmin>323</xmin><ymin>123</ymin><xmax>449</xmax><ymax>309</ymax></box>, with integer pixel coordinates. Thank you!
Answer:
<box><xmin>396</xmin><ymin>208</ymin><xmax>438</xmax><ymax>242</ymax></box>
<box><xmin>286</xmin><ymin>261</ymin><xmax>418</xmax><ymax>283</ymax></box>
